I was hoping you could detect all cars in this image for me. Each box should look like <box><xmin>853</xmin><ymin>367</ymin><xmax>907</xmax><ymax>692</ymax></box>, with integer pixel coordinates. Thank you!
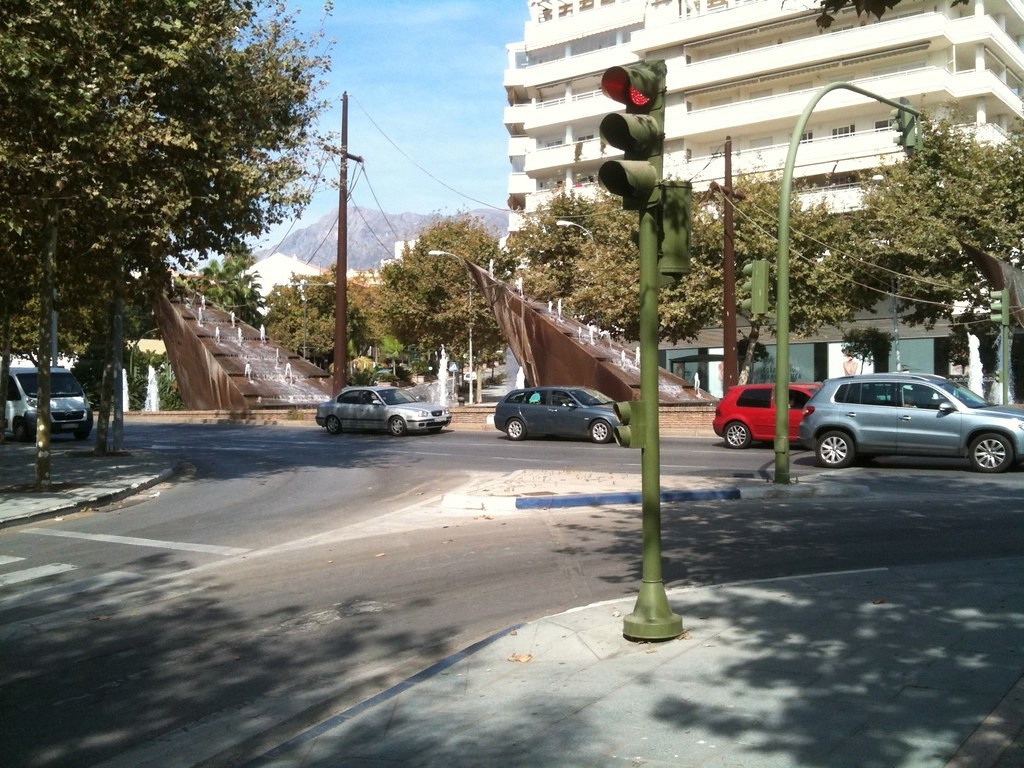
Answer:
<box><xmin>712</xmin><ymin>383</ymin><xmax>821</xmax><ymax>449</ymax></box>
<box><xmin>314</xmin><ymin>386</ymin><xmax>452</xmax><ymax>436</ymax></box>
<box><xmin>494</xmin><ymin>386</ymin><xmax>624</xmax><ymax>444</ymax></box>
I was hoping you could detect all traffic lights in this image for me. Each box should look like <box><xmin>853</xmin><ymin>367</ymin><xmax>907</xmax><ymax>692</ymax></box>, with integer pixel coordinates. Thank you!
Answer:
<box><xmin>597</xmin><ymin>57</ymin><xmax>668</xmax><ymax>212</ymax></box>
<box><xmin>989</xmin><ymin>289</ymin><xmax>1010</xmax><ymax>326</ymax></box>
<box><xmin>890</xmin><ymin>96</ymin><xmax>920</xmax><ymax>154</ymax></box>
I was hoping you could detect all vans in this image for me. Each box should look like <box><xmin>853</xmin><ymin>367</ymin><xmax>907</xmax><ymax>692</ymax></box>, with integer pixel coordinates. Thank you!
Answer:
<box><xmin>0</xmin><ymin>367</ymin><xmax>94</xmax><ymax>442</ymax></box>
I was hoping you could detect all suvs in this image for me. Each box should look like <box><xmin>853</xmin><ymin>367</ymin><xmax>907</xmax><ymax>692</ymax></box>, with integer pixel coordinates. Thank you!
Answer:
<box><xmin>798</xmin><ymin>372</ymin><xmax>1024</xmax><ymax>473</ymax></box>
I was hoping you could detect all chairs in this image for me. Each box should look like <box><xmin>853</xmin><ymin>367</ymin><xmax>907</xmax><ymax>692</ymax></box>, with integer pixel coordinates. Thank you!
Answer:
<box><xmin>553</xmin><ymin>401</ymin><xmax>563</xmax><ymax>406</ymax></box>
